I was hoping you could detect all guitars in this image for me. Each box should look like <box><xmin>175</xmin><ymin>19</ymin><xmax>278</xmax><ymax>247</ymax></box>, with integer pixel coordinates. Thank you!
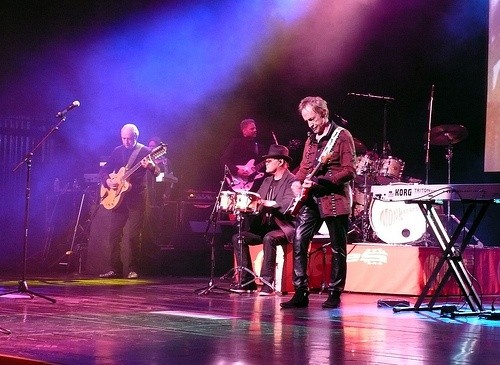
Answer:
<box><xmin>100</xmin><ymin>141</ymin><xmax>169</xmax><ymax>210</ymax></box>
<box><xmin>224</xmin><ymin>138</ymin><xmax>301</xmax><ymax>192</ymax></box>
<box><xmin>283</xmin><ymin>151</ymin><xmax>334</xmax><ymax>215</ymax></box>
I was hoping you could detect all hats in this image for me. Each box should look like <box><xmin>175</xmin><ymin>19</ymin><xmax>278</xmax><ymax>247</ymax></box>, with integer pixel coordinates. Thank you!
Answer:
<box><xmin>261</xmin><ymin>144</ymin><xmax>294</xmax><ymax>161</ymax></box>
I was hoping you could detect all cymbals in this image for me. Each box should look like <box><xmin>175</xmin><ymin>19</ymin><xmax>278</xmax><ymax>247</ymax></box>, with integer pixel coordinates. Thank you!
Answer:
<box><xmin>429</xmin><ymin>124</ymin><xmax>465</xmax><ymax>146</ymax></box>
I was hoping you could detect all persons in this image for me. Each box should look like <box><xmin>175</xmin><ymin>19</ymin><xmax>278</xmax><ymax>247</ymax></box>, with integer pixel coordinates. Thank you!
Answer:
<box><xmin>222</xmin><ymin>116</ymin><xmax>265</xmax><ymax>194</ymax></box>
<box><xmin>144</xmin><ymin>135</ymin><xmax>175</xmax><ymax>214</ymax></box>
<box><xmin>98</xmin><ymin>122</ymin><xmax>161</xmax><ymax>278</ymax></box>
<box><xmin>279</xmin><ymin>95</ymin><xmax>357</xmax><ymax>309</ymax></box>
<box><xmin>229</xmin><ymin>142</ymin><xmax>297</xmax><ymax>295</ymax></box>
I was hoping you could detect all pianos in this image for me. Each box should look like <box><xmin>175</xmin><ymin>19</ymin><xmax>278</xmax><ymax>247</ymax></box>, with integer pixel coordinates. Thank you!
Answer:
<box><xmin>370</xmin><ymin>184</ymin><xmax>500</xmax><ymax>312</ymax></box>
<box><xmin>85</xmin><ymin>173</ymin><xmax>179</xmax><ymax>249</ymax></box>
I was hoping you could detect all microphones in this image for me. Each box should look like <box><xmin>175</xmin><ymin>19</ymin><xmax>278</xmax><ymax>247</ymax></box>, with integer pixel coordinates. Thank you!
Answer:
<box><xmin>225</xmin><ymin>164</ymin><xmax>234</xmax><ymax>186</ymax></box>
<box><xmin>55</xmin><ymin>101</ymin><xmax>80</xmax><ymax>117</ymax></box>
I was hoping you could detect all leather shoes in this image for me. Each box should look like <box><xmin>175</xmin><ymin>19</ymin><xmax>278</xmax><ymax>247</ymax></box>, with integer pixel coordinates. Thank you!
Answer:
<box><xmin>100</xmin><ymin>270</ymin><xmax>122</xmax><ymax>277</ymax></box>
<box><xmin>322</xmin><ymin>291</ymin><xmax>342</xmax><ymax>308</ymax></box>
<box><xmin>127</xmin><ymin>271</ymin><xmax>137</xmax><ymax>278</ymax></box>
<box><xmin>280</xmin><ymin>290</ymin><xmax>310</xmax><ymax>307</ymax></box>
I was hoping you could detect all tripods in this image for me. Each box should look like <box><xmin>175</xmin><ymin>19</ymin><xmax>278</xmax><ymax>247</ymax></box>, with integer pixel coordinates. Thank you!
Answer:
<box><xmin>195</xmin><ymin>176</ymin><xmax>237</xmax><ymax>298</ymax></box>
<box><xmin>1</xmin><ymin>118</ymin><xmax>68</xmax><ymax>305</ymax></box>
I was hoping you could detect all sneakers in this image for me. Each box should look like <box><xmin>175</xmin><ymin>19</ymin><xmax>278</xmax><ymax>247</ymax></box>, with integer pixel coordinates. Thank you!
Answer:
<box><xmin>259</xmin><ymin>280</ymin><xmax>274</xmax><ymax>295</ymax></box>
<box><xmin>231</xmin><ymin>283</ymin><xmax>257</xmax><ymax>294</ymax></box>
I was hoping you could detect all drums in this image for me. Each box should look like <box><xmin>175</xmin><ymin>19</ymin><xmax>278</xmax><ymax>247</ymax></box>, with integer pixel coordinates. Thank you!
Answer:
<box><xmin>376</xmin><ymin>158</ymin><xmax>406</xmax><ymax>184</ymax></box>
<box><xmin>352</xmin><ymin>187</ymin><xmax>371</xmax><ymax>204</ymax></box>
<box><xmin>352</xmin><ymin>156</ymin><xmax>378</xmax><ymax>183</ymax></box>
<box><xmin>218</xmin><ymin>189</ymin><xmax>263</xmax><ymax>215</ymax></box>
<box><xmin>368</xmin><ymin>181</ymin><xmax>444</xmax><ymax>244</ymax></box>
